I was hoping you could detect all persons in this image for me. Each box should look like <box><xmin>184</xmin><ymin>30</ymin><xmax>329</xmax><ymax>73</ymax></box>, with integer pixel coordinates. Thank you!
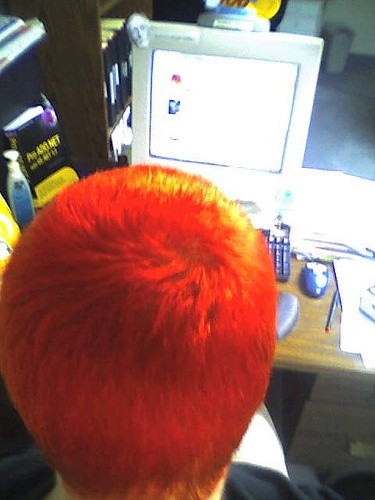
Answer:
<box><xmin>0</xmin><ymin>164</ymin><xmax>375</xmax><ymax>500</ymax></box>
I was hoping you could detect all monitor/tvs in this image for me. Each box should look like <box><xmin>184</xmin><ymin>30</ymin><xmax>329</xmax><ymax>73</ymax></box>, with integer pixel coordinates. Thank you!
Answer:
<box><xmin>131</xmin><ymin>21</ymin><xmax>324</xmax><ymax>205</ymax></box>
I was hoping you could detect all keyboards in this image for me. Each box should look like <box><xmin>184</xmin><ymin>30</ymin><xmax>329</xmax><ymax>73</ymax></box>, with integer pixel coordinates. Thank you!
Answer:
<box><xmin>250</xmin><ymin>223</ymin><xmax>291</xmax><ymax>282</ymax></box>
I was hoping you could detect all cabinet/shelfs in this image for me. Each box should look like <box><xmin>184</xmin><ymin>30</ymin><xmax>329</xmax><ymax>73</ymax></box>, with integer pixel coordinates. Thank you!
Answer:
<box><xmin>0</xmin><ymin>0</ymin><xmax>154</xmax><ymax>170</ymax></box>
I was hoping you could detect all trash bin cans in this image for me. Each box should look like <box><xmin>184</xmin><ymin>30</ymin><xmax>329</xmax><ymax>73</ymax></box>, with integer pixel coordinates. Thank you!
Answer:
<box><xmin>323</xmin><ymin>22</ymin><xmax>355</xmax><ymax>74</ymax></box>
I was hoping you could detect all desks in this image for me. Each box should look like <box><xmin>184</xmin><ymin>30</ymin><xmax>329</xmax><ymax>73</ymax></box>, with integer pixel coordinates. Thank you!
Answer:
<box><xmin>262</xmin><ymin>166</ymin><xmax>375</xmax><ymax>455</ymax></box>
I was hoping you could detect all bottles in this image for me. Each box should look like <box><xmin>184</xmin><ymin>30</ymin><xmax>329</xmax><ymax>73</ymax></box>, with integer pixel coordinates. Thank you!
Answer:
<box><xmin>0</xmin><ymin>193</ymin><xmax>23</xmax><ymax>279</ymax></box>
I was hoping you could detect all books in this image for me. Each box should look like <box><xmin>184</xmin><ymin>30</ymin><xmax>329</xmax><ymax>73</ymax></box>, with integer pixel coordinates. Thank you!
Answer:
<box><xmin>0</xmin><ymin>102</ymin><xmax>76</xmax><ymax>198</ymax></box>
<box><xmin>99</xmin><ymin>18</ymin><xmax>133</xmax><ymax>127</ymax></box>
<box><xmin>0</xmin><ymin>14</ymin><xmax>46</xmax><ymax>77</ymax></box>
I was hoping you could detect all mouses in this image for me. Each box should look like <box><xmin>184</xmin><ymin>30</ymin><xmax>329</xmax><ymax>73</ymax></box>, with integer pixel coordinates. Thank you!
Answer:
<box><xmin>301</xmin><ymin>261</ymin><xmax>329</xmax><ymax>298</ymax></box>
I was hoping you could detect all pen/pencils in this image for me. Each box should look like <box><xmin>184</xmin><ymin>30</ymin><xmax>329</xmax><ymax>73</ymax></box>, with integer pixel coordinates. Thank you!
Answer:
<box><xmin>326</xmin><ymin>290</ymin><xmax>340</xmax><ymax>332</ymax></box>
